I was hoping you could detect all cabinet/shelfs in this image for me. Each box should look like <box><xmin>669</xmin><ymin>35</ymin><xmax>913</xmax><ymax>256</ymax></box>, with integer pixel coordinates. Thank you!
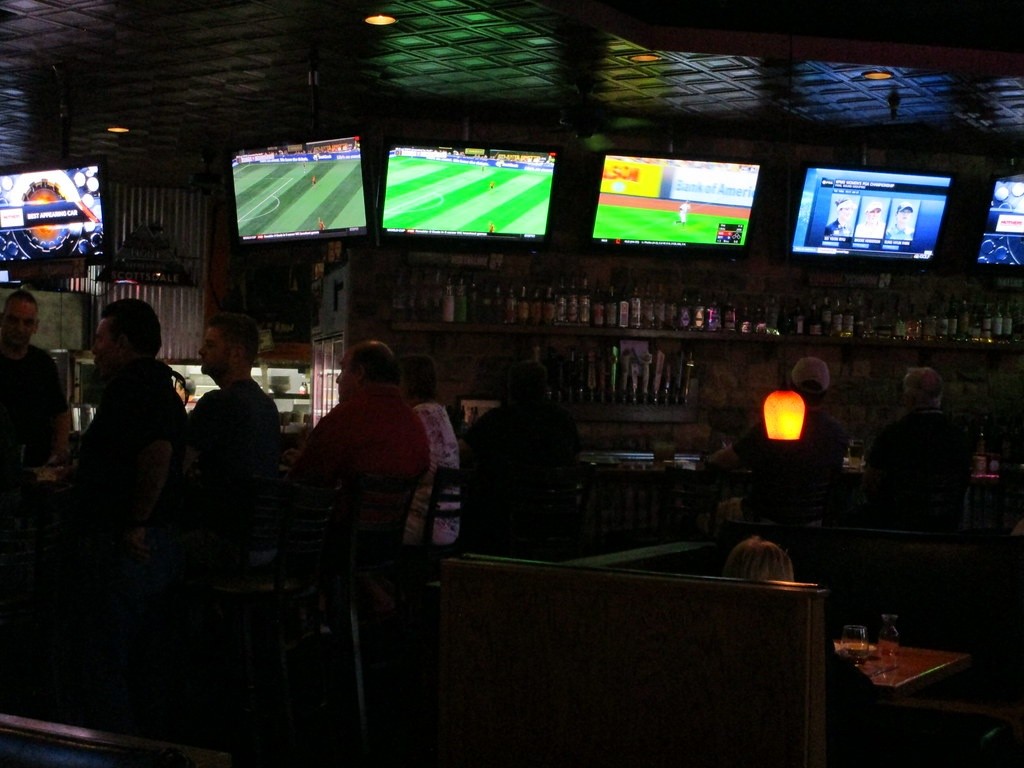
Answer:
<box><xmin>389</xmin><ymin>319</ymin><xmax>1024</xmax><ymax>426</ymax></box>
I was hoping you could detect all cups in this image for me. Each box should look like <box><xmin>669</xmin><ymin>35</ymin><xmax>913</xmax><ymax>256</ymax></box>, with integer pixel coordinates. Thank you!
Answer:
<box><xmin>840</xmin><ymin>626</ymin><xmax>870</xmax><ymax>667</ymax></box>
<box><xmin>849</xmin><ymin>439</ymin><xmax>868</xmax><ymax>468</ymax></box>
<box><xmin>879</xmin><ymin>614</ymin><xmax>901</xmax><ymax>664</ymax></box>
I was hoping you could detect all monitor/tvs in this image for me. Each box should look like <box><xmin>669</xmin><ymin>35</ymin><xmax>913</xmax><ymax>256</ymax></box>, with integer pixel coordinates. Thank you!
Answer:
<box><xmin>375</xmin><ymin>137</ymin><xmax>564</xmax><ymax>254</ymax></box>
<box><xmin>784</xmin><ymin>160</ymin><xmax>959</xmax><ymax>274</ymax></box>
<box><xmin>585</xmin><ymin>149</ymin><xmax>766</xmax><ymax>255</ymax></box>
<box><xmin>231</xmin><ymin>134</ymin><xmax>369</xmax><ymax>247</ymax></box>
<box><xmin>973</xmin><ymin>173</ymin><xmax>1024</xmax><ymax>269</ymax></box>
<box><xmin>0</xmin><ymin>153</ymin><xmax>111</xmax><ymax>270</ymax></box>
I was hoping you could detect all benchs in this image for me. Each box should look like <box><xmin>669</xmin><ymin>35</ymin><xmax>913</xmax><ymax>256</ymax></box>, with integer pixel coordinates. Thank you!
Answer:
<box><xmin>436</xmin><ymin>550</ymin><xmax>826</xmax><ymax>768</ymax></box>
<box><xmin>716</xmin><ymin>518</ymin><xmax>1024</xmax><ymax>768</ymax></box>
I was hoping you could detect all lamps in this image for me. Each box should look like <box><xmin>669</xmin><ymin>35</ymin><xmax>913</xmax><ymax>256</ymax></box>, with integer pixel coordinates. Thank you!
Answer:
<box><xmin>761</xmin><ymin>36</ymin><xmax>808</xmax><ymax>443</ymax></box>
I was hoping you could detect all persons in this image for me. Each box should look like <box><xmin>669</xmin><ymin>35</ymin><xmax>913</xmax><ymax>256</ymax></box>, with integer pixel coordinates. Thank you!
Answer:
<box><xmin>857</xmin><ymin>367</ymin><xmax>980</xmax><ymax>534</ymax></box>
<box><xmin>187</xmin><ymin>313</ymin><xmax>282</xmax><ymax>655</ymax></box>
<box><xmin>286</xmin><ymin>340</ymin><xmax>461</xmax><ymax>640</ymax></box>
<box><xmin>74</xmin><ymin>298</ymin><xmax>187</xmax><ymax>738</ymax></box>
<box><xmin>458</xmin><ymin>359</ymin><xmax>578</xmax><ymax>559</ymax></box>
<box><xmin>884</xmin><ymin>202</ymin><xmax>915</xmax><ymax>240</ymax></box>
<box><xmin>0</xmin><ymin>290</ymin><xmax>71</xmax><ymax>499</ymax></box>
<box><xmin>723</xmin><ymin>536</ymin><xmax>872</xmax><ymax>700</ymax></box>
<box><xmin>825</xmin><ymin>198</ymin><xmax>857</xmax><ymax>237</ymax></box>
<box><xmin>696</xmin><ymin>356</ymin><xmax>851</xmax><ymax>526</ymax></box>
<box><xmin>854</xmin><ymin>201</ymin><xmax>886</xmax><ymax>240</ymax></box>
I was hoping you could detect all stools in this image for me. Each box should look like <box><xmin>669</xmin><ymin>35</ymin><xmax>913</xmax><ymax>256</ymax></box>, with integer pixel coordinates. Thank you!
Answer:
<box><xmin>0</xmin><ymin>465</ymin><xmax>968</xmax><ymax>768</ymax></box>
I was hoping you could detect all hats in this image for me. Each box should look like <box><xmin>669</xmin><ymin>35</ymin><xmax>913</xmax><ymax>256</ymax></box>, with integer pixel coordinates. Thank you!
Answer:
<box><xmin>792</xmin><ymin>357</ymin><xmax>831</xmax><ymax>394</ymax></box>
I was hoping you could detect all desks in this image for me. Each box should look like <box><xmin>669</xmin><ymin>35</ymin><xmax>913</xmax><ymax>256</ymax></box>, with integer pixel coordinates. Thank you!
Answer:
<box><xmin>833</xmin><ymin>638</ymin><xmax>972</xmax><ymax>702</ymax></box>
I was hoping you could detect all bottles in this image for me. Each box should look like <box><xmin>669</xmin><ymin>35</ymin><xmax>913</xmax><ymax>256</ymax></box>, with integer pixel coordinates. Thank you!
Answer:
<box><xmin>963</xmin><ymin>425</ymin><xmax>1019</xmax><ymax>463</ymax></box>
<box><xmin>390</xmin><ymin>263</ymin><xmax>1024</xmax><ymax>340</ymax></box>
<box><xmin>541</xmin><ymin>345</ymin><xmax>695</xmax><ymax>405</ymax></box>
